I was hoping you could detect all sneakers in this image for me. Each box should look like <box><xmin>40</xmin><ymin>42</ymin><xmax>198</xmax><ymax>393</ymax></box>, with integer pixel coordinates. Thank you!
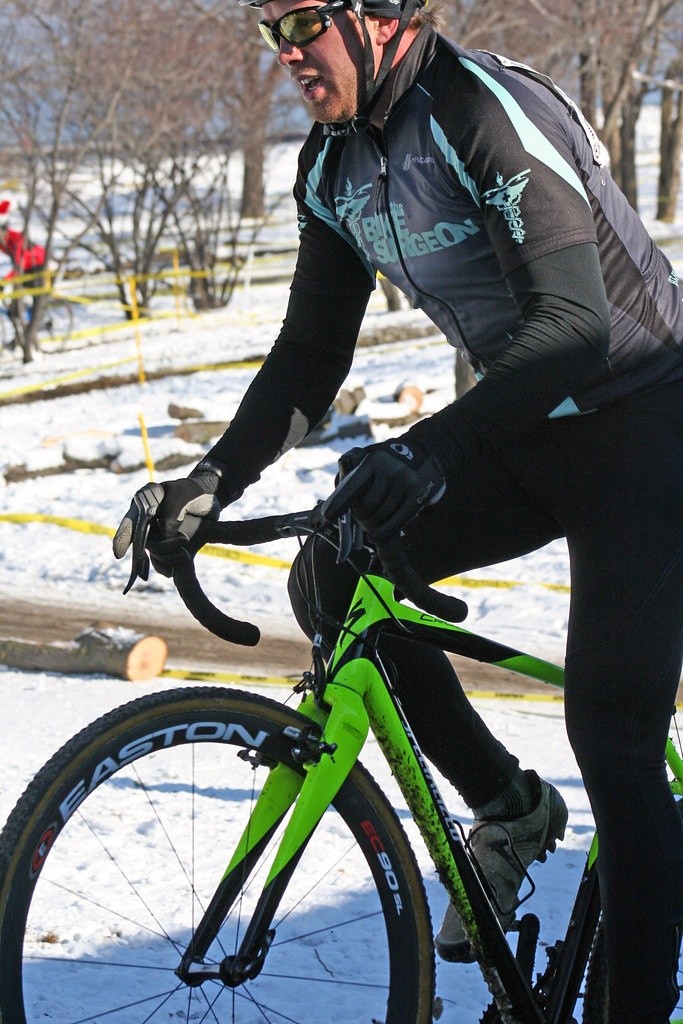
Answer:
<box><xmin>439</xmin><ymin>772</ymin><xmax>571</xmax><ymax>963</ymax></box>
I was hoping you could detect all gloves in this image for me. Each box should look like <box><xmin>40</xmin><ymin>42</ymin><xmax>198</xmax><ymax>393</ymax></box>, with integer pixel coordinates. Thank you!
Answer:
<box><xmin>113</xmin><ymin>466</ymin><xmax>235</xmax><ymax>581</ymax></box>
<box><xmin>321</xmin><ymin>433</ymin><xmax>448</xmax><ymax>541</ymax></box>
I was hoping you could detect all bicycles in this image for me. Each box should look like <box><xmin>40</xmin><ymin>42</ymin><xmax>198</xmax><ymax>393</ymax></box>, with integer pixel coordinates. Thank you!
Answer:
<box><xmin>0</xmin><ymin>452</ymin><xmax>683</xmax><ymax>1024</ymax></box>
<box><xmin>0</xmin><ymin>278</ymin><xmax>75</xmax><ymax>356</ymax></box>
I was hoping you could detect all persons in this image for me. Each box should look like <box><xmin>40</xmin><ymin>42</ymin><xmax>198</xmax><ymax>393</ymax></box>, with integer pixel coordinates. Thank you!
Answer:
<box><xmin>112</xmin><ymin>0</ymin><xmax>683</xmax><ymax>1024</ymax></box>
<box><xmin>0</xmin><ymin>202</ymin><xmax>51</xmax><ymax>364</ymax></box>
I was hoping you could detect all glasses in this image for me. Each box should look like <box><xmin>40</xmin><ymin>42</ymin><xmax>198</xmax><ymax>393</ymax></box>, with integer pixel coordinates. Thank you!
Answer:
<box><xmin>256</xmin><ymin>1</ymin><xmax>343</xmax><ymax>54</ymax></box>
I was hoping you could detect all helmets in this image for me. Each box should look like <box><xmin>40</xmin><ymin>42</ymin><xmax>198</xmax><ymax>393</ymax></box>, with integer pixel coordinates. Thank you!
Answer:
<box><xmin>238</xmin><ymin>0</ymin><xmax>428</xmax><ymax>17</ymax></box>
